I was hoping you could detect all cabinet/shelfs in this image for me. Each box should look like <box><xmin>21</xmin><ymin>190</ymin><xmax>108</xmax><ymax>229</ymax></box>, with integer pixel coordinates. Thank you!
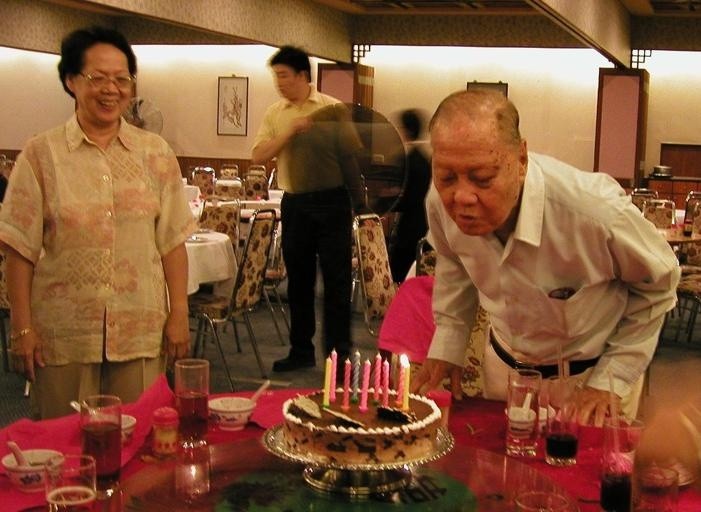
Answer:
<box><xmin>647</xmin><ymin>172</ymin><xmax>699</xmax><ymax>208</ymax></box>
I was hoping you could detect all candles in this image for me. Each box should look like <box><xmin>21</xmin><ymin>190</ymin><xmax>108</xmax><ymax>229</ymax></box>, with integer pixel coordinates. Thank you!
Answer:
<box><xmin>320</xmin><ymin>349</ymin><xmax>416</xmax><ymax>419</ymax></box>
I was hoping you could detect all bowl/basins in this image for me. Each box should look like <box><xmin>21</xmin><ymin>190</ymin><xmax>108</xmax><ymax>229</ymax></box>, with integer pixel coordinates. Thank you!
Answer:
<box><xmin>506</xmin><ymin>404</ymin><xmax>556</xmax><ymax>435</ymax></box>
<box><xmin>1</xmin><ymin>449</ymin><xmax>64</xmax><ymax>493</ymax></box>
<box><xmin>89</xmin><ymin>414</ymin><xmax>136</xmax><ymax>444</ymax></box>
<box><xmin>206</xmin><ymin>397</ymin><xmax>256</xmax><ymax>431</ymax></box>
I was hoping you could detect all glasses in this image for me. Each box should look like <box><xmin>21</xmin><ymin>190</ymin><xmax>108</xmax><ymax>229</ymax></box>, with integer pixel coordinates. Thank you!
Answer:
<box><xmin>78</xmin><ymin>70</ymin><xmax>135</xmax><ymax>91</ymax></box>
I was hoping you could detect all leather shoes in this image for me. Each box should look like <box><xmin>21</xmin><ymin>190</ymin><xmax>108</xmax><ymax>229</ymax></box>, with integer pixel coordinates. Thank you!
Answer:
<box><xmin>272</xmin><ymin>355</ymin><xmax>315</xmax><ymax>372</ymax></box>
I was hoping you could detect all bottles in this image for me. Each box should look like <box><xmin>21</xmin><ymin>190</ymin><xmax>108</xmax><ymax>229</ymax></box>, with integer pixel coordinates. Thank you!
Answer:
<box><xmin>151</xmin><ymin>405</ymin><xmax>179</xmax><ymax>456</ymax></box>
<box><xmin>684</xmin><ymin>220</ymin><xmax>693</xmax><ymax>237</ymax></box>
<box><xmin>428</xmin><ymin>391</ymin><xmax>453</xmax><ymax>434</ymax></box>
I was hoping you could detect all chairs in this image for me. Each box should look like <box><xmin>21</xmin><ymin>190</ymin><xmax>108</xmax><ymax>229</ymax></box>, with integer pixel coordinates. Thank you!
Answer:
<box><xmin>376</xmin><ymin>277</ymin><xmax>493</xmax><ymax>397</ymax></box>
<box><xmin>346</xmin><ymin>209</ymin><xmax>394</xmax><ymax>336</ymax></box>
<box><xmin>4</xmin><ymin>154</ymin><xmax>295</xmax><ymax>392</ymax></box>
<box><xmin>624</xmin><ymin>185</ymin><xmax>701</xmax><ymax>360</ymax></box>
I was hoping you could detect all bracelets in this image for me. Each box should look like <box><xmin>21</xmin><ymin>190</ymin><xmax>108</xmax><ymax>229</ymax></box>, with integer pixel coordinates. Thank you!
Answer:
<box><xmin>5</xmin><ymin>327</ymin><xmax>32</xmax><ymax>343</ymax></box>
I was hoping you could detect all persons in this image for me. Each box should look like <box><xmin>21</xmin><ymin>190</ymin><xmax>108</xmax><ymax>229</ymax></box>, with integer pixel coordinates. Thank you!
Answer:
<box><xmin>251</xmin><ymin>45</ymin><xmax>371</xmax><ymax>383</ymax></box>
<box><xmin>0</xmin><ymin>23</ymin><xmax>202</xmax><ymax>413</ymax></box>
<box><xmin>410</xmin><ymin>86</ymin><xmax>682</xmax><ymax>431</ymax></box>
<box><xmin>381</xmin><ymin>108</ymin><xmax>435</xmax><ymax>280</ymax></box>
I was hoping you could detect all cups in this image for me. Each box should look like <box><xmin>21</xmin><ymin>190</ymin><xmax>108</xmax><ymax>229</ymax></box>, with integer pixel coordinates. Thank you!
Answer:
<box><xmin>632</xmin><ymin>469</ymin><xmax>679</xmax><ymax>511</ymax></box>
<box><xmin>512</xmin><ymin>491</ymin><xmax>568</xmax><ymax>511</ymax></box>
<box><xmin>44</xmin><ymin>455</ymin><xmax>98</xmax><ymax>512</ymax></box>
<box><xmin>174</xmin><ymin>358</ymin><xmax>211</xmax><ymax>440</ymax></box>
<box><xmin>544</xmin><ymin>376</ymin><xmax>583</xmax><ymax>467</ymax></box>
<box><xmin>79</xmin><ymin>396</ymin><xmax>123</xmax><ymax>499</ymax></box>
<box><xmin>176</xmin><ymin>439</ymin><xmax>210</xmax><ymax>502</ymax></box>
<box><xmin>599</xmin><ymin>417</ymin><xmax>644</xmax><ymax>511</ymax></box>
<box><xmin>504</xmin><ymin>369</ymin><xmax>539</xmax><ymax>459</ymax></box>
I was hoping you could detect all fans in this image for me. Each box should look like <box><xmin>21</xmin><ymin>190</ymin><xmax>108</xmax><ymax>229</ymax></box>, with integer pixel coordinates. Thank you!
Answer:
<box><xmin>118</xmin><ymin>96</ymin><xmax>164</xmax><ymax>137</ymax></box>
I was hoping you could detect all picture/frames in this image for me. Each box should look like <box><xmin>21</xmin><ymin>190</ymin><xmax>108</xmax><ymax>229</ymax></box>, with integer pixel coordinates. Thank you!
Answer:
<box><xmin>216</xmin><ymin>74</ymin><xmax>249</xmax><ymax>138</ymax></box>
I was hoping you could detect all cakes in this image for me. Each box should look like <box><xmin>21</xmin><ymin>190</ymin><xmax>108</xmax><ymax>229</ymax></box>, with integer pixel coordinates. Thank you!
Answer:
<box><xmin>281</xmin><ymin>387</ymin><xmax>442</xmax><ymax>464</ymax></box>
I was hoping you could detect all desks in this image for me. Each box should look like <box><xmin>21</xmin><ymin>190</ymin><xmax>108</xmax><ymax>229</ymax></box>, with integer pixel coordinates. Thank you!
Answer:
<box><xmin>0</xmin><ymin>381</ymin><xmax>700</xmax><ymax>512</ymax></box>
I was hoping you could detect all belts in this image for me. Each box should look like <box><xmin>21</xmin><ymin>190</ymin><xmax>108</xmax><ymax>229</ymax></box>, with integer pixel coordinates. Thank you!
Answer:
<box><xmin>489</xmin><ymin>327</ymin><xmax>600</xmax><ymax>380</ymax></box>
